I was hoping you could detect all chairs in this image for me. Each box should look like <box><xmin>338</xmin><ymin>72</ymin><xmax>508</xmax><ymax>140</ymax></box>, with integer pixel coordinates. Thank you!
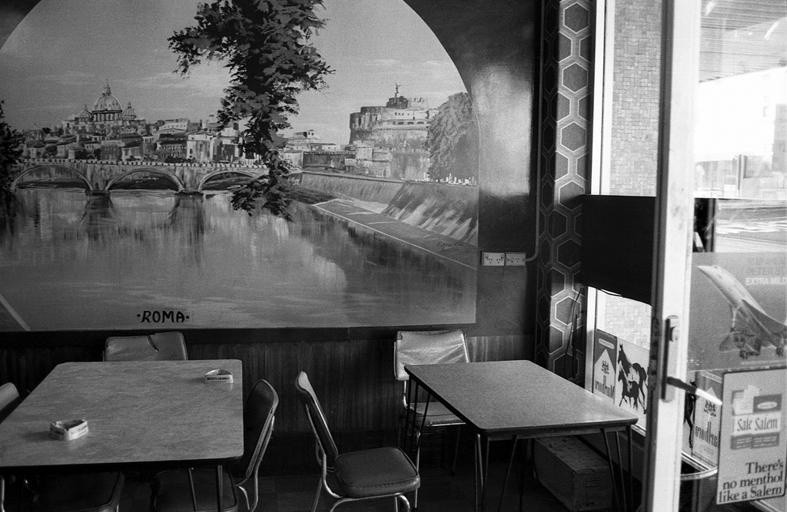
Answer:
<box><xmin>135</xmin><ymin>376</ymin><xmax>280</xmax><ymax>512</ymax></box>
<box><xmin>393</xmin><ymin>329</ymin><xmax>474</xmax><ymax>509</ymax></box>
<box><xmin>294</xmin><ymin>370</ymin><xmax>421</xmax><ymax>512</ymax></box>
<box><xmin>0</xmin><ymin>382</ymin><xmax>126</xmax><ymax>512</ymax></box>
<box><xmin>103</xmin><ymin>332</ymin><xmax>188</xmax><ymax>361</ymax></box>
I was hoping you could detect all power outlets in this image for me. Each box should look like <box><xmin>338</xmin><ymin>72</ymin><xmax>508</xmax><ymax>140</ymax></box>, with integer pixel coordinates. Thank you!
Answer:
<box><xmin>480</xmin><ymin>250</ymin><xmax>529</xmax><ymax>268</ymax></box>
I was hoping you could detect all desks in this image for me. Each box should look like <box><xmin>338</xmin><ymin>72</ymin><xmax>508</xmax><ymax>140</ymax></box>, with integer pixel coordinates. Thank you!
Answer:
<box><xmin>404</xmin><ymin>360</ymin><xmax>639</xmax><ymax>511</ymax></box>
<box><xmin>0</xmin><ymin>358</ymin><xmax>245</xmax><ymax>512</ymax></box>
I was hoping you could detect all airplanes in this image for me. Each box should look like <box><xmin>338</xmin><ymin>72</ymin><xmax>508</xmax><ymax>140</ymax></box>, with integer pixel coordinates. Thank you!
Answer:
<box><xmin>696</xmin><ymin>264</ymin><xmax>787</xmax><ymax>359</ymax></box>
